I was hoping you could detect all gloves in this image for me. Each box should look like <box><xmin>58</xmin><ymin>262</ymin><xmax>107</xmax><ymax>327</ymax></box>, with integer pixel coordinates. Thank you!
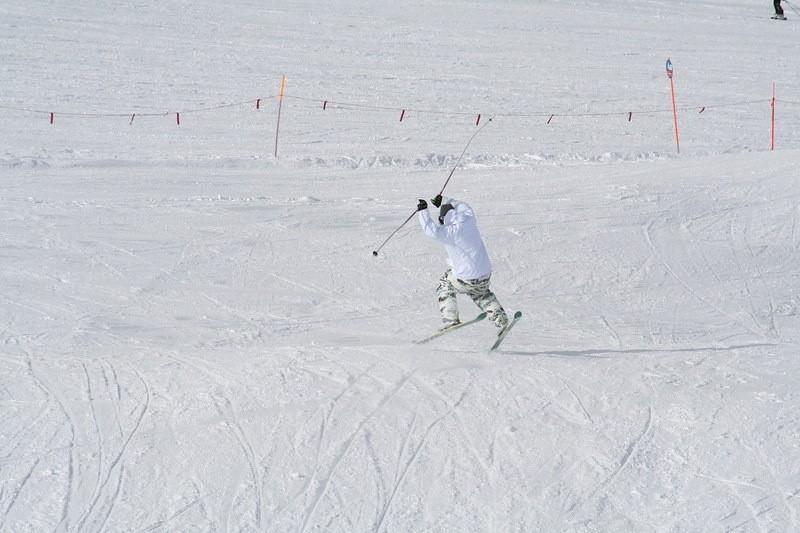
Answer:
<box><xmin>417</xmin><ymin>199</ymin><xmax>427</xmax><ymax>210</ymax></box>
<box><xmin>431</xmin><ymin>195</ymin><xmax>442</xmax><ymax>207</ymax></box>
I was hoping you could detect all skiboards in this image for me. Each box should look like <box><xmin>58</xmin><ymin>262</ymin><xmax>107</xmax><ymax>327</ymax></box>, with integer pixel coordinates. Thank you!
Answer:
<box><xmin>416</xmin><ymin>312</ymin><xmax>523</xmax><ymax>350</ymax></box>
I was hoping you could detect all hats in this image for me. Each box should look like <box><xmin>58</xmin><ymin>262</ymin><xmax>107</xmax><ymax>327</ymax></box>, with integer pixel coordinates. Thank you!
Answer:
<box><xmin>440</xmin><ymin>204</ymin><xmax>454</xmax><ymax>220</ymax></box>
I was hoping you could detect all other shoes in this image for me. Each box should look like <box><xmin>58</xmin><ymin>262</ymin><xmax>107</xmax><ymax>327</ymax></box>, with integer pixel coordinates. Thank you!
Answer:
<box><xmin>440</xmin><ymin>319</ymin><xmax>461</xmax><ymax>331</ymax></box>
<box><xmin>497</xmin><ymin>321</ymin><xmax>509</xmax><ymax>335</ymax></box>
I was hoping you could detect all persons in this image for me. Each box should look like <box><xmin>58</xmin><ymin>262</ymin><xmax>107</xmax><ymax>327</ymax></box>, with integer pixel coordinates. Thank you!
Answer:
<box><xmin>417</xmin><ymin>195</ymin><xmax>509</xmax><ymax>337</ymax></box>
<box><xmin>771</xmin><ymin>0</ymin><xmax>784</xmax><ymax>20</ymax></box>
